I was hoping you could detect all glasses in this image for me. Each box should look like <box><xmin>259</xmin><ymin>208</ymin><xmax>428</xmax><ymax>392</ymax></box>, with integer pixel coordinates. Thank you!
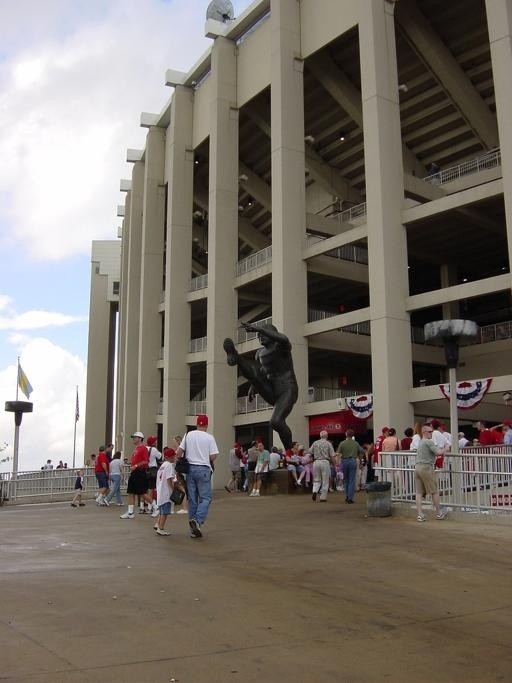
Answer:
<box><xmin>426</xmin><ymin>430</ymin><xmax>434</xmax><ymax>433</ymax></box>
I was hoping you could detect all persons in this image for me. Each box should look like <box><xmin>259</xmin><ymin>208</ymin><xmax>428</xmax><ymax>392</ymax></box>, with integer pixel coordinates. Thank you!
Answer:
<box><xmin>95</xmin><ymin>444</ymin><xmax>126</xmax><ymax>506</ymax></box>
<box><xmin>373</xmin><ymin>422</ymin><xmax>423</xmax><ymax>494</ymax></box>
<box><xmin>458</xmin><ymin>421</ymin><xmax>511</xmax><ymax>492</ymax></box>
<box><xmin>56</xmin><ymin>460</ymin><xmax>67</xmax><ymax>469</ymax></box>
<box><xmin>120</xmin><ymin>432</ymin><xmax>160</xmax><ymax>518</ymax></box>
<box><xmin>222</xmin><ymin>320</ymin><xmax>299</xmax><ymax>451</ymax></box>
<box><xmin>337</xmin><ymin>429</ymin><xmax>363</xmax><ymax>503</ymax></box>
<box><xmin>139</xmin><ymin>435</ymin><xmax>163</xmax><ymax>513</ymax></box>
<box><xmin>225</xmin><ymin>441</ymin><xmax>313</xmax><ymax>497</ymax></box>
<box><xmin>327</xmin><ymin>439</ymin><xmax>340</xmax><ymax>492</ymax></box>
<box><xmin>430</xmin><ymin>420</ymin><xmax>451</xmax><ymax>495</ymax></box>
<box><xmin>153</xmin><ymin>447</ymin><xmax>177</xmax><ymax>537</ymax></box>
<box><xmin>86</xmin><ymin>453</ymin><xmax>96</xmax><ymax>467</ymax></box>
<box><xmin>176</xmin><ymin>435</ymin><xmax>184</xmax><ymax>457</ymax></box>
<box><xmin>41</xmin><ymin>467</ymin><xmax>44</xmax><ymax>470</ymax></box>
<box><xmin>70</xmin><ymin>470</ymin><xmax>85</xmax><ymax>506</ymax></box>
<box><xmin>44</xmin><ymin>459</ymin><xmax>53</xmax><ymax>470</ymax></box>
<box><xmin>308</xmin><ymin>430</ymin><xmax>336</xmax><ymax>502</ymax></box>
<box><xmin>175</xmin><ymin>414</ymin><xmax>219</xmax><ymax>539</ymax></box>
<box><xmin>414</xmin><ymin>422</ymin><xmax>449</xmax><ymax>520</ymax></box>
<box><xmin>356</xmin><ymin>443</ymin><xmax>375</xmax><ymax>492</ymax></box>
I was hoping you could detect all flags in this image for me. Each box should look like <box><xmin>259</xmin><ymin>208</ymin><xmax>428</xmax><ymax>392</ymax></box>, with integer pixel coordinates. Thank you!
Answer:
<box><xmin>18</xmin><ymin>364</ymin><xmax>34</xmax><ymax>400</ymax></box>
<box><xmin>76</xmin><ymin>392</ymin><xmax>80</xmax><ymax>423</ymax></box>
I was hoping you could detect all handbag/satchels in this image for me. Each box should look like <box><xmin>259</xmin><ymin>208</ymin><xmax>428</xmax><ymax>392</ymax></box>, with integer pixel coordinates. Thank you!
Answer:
<box><xmin>171</xmin><ymin>487</ymin><xmax>185</xmax><ymax>504</ymax></box>
<box><xmin>176</xmin><ymin>457</ymin><xmax>189</xmax><ymax>473</ymax></box>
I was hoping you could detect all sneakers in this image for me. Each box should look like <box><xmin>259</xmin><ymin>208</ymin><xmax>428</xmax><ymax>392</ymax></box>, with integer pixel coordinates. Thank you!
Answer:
<box><xmin>435</xmin><ymin>511</ymin><xmax>447</xmax><ymax>519</ymax></box>
<box><xmin>224</xmin><ymin>483</ymin><xmax>260</xmax><ymax>496</ymax></box>
<box><xmin>345</xmin><ymin>496</ymin><xmax>354</xmax><ymax>504</ymax></box>
<box><xmin>120</xmin><ymin>512</ymin><xmax>135</xmax><ymax>518</ymax></box>
<box><xmin>295</xmin><ymin>478</ymin><xmax>303</xmax><ymax>486</ymax></box>
<box><xmin>80</xmin><ymin>503</ymin><xmax>85</xmax><ymax>506</ymax></box>
<box><xmin>154</xmin><ymin>523</ymin><xmax>171</xmax><ymax>535</ymax></box>
<box><xmin>418</xmin><ymin>513</ymin><xmax>425</xmax><ymax>522</ymax></box>
<box><xmin>95</xmin><ymin>492</ymin><xmax>124</xmax><ymax>506</ymax></box>
<box><xmin>312</xmin><ymin>492</ymin><xmax>317</xmax><ymax>501</ymax></box>
<box><xmin>138</xmin><ymin>506</ymin><xmax>160</xmax><ymax>517</ymax></box>
<box><xmin>71</xmin><ymin>503</ymin><xmax>77</xmax><ymax>507</ymax></box>
<box><xmin>329</xmin><ymin>484</ymin><xmax>345</xmax><ymax>492</ymax></box>
<box><xmin>189</xmin><ymin>519</ymin><xmax>203</xmax><ymax>538</ymax></box>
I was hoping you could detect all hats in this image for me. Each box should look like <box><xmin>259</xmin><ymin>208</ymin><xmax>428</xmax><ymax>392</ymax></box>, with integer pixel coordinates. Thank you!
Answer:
<box><xmin>382</xmin><ymin>427</ymin><xmax>389</xmax><ymax>433</ymax></box>
<box><xmin>164</xmin><ymin>447</ymin><xmax>176</xmax><ymax>459</ymax></box>
<box><xmin>131</xmin><ymin>432</ymin><xmax>144</xmax><ymax>439</ymax></box>
<box><xmin>431</xmin><ymin>419</ymin><xmax>440</xmax><ymax>428</ymax></box>
<box><xmin>147</xmin><ymin>436</ymin><xmax>157</xmax><ymax>445</ymax></box>
<box><xmin>197</xmin><ymin>416</ymin><xmax>208</xmax><ymax>425</ymax></box>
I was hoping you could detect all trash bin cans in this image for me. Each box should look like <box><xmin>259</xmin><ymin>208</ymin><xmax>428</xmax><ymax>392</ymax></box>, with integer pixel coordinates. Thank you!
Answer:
<box><xmin>364</xmin><ymin>481</ymin><xmax>392</xmax><ymax>517</ymax></box>
